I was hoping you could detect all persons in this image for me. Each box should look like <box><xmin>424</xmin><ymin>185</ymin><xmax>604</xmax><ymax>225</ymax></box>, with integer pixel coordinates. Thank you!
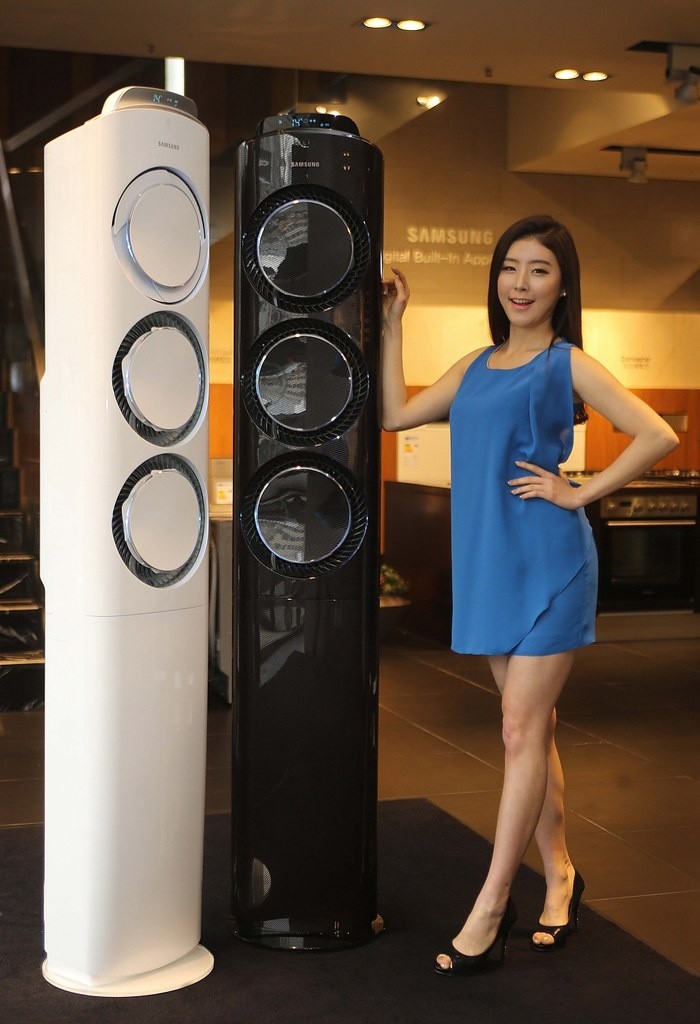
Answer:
<box><xmin>385</xmin><ymin>216</ymin><xmax>678</xmax><ymax>977</ymax></box>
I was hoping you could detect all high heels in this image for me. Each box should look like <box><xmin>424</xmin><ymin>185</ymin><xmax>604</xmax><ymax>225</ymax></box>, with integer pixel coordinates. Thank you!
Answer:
<box><xmin>434</xmin><ymin>887</ymin><xmax>518</xmax><ymax>977</ymax></box>
<box><xmin>527</xmin><ymin>871</ymin><xmax>586</xmax><ymax>952</ymax></box>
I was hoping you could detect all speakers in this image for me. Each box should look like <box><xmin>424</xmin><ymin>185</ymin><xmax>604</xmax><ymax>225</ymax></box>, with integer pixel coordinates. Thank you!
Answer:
<box><xmin>569</xmin><ymin>476</ymin><xmax>700</xmax><ymax>613</ymax></box>
